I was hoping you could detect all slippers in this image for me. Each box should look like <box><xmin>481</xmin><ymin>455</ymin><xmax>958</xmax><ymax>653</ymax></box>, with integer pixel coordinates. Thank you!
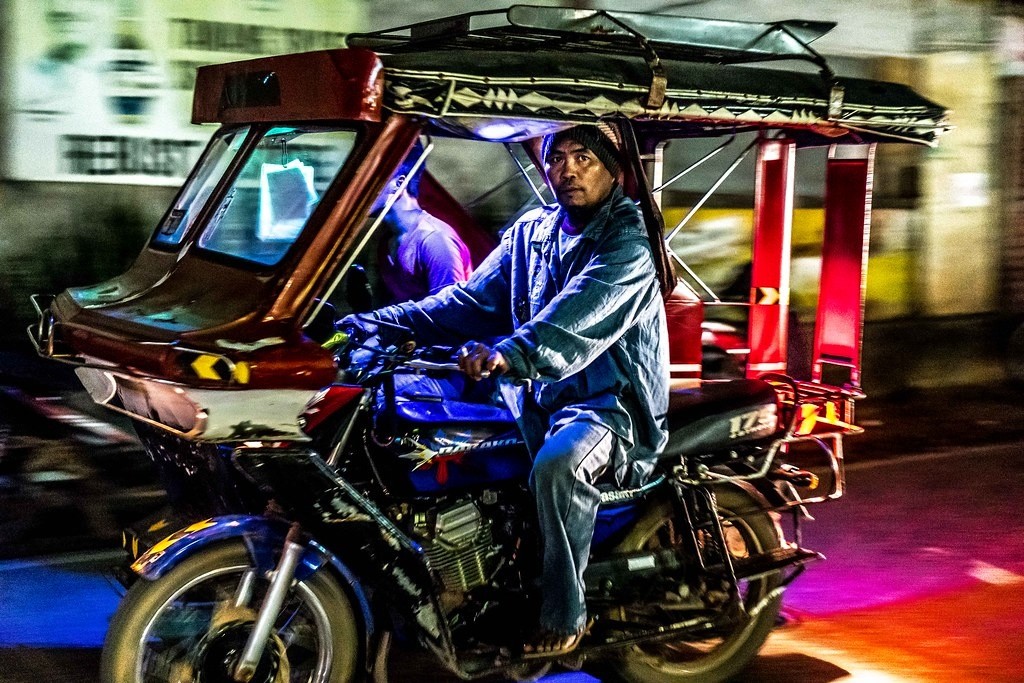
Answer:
<box><xmin>519</xmin><ymin>614</ymin><xmax>596</xmax><ymax>660</ymax></box>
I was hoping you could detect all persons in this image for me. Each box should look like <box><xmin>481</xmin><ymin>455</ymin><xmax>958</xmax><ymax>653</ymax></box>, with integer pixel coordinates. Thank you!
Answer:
<box><xmin>368</xmin><ymin>136</ymin><xmax>472</xmax><ymax>305</ymax></box>
<box><xmin>333</xmin><ymin>118</ymin><xmax>670</xmax><ymax>658</ymax></box>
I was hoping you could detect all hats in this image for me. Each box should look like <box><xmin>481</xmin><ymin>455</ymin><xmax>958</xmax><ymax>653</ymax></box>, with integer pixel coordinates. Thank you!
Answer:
<box><xmin>542</xmin><ymin>120</ymin><xmax>625</xmax><ymax>182</ymax></box>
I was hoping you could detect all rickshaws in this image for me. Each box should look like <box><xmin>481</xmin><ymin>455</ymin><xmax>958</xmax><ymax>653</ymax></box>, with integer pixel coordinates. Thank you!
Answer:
<box><xmin>26</xmin><ymin>1</ymin><xmax>954</xmax><ymax>683</ymax></box>
